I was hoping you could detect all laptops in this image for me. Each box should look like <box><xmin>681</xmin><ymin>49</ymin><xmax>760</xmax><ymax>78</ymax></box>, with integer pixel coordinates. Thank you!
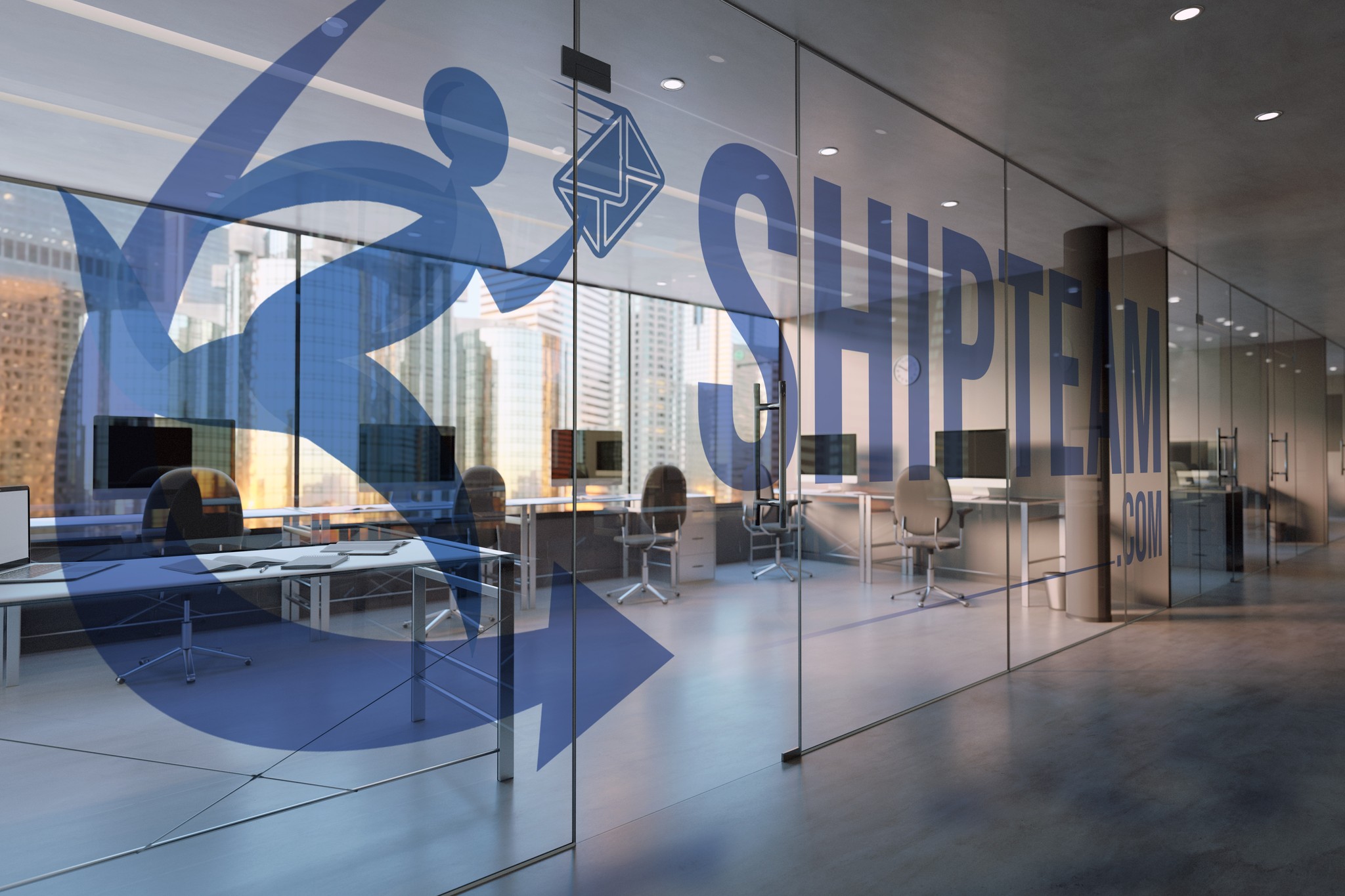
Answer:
<box><xmin>0</xmin><ymin>486</ymin><xmax>120</xmax><ymax>584</ymax></box>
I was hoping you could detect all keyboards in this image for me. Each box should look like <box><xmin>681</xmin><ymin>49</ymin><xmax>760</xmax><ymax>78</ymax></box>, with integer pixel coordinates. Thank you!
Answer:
<box><xmin>802</xmin><ymin>489</ymin><xmax>830</xmax><ymax>493</ymax></box>
<box><xmin>579</xmin><ymin>495</ymin><xmax>627</xmax><ymax>501</ymax></box>
<box><xmin>399</xmin><ymin>502</ymin><xmax>458</xmax><ymax>508</ymax></box>
<box><xmin>951</xmin><ymin>495</ymin><xmax>980</xmax><ymax>501</ymax></box>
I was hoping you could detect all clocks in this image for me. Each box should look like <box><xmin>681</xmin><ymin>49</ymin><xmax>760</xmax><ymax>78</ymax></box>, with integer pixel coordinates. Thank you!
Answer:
<box><xmin>893</xmin><ymin>354</ymin><xmax>921</xmax><ymax>387</ymax></box>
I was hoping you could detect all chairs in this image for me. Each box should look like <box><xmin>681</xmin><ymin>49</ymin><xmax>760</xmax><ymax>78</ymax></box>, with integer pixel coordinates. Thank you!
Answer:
<box><xmin>114</xmin><ymin>467</ymin><xmax>254</xmax><ymax>688</ymax></box>
<box><xmin>890</xmin><ymin>465</ymin><xmax>974</xmax><ymax>606</ymax></box>
<box><xmin>403</xmin><ymin>466</ymin><xmax>509</xmax><ymax>638</ymax></box>
<box><xmin>743</xmin><ymin>463</ymin><xmax>813</xmax><ymax>581</ymax></box>
<box><xmin>606</xmin><ymin>465</ymin><xmax>687</xmax><ymax>604</ymax></box>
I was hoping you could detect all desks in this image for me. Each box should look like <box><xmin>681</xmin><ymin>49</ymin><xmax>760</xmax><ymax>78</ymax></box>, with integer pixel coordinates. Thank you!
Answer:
<box><xmin>866</xmin><ymin>495</ymin><xmax>1062</xmax><ymax>608</ymax></box>
<box><xmin>773</xmin><ymin>487</ymin><xmax>870</xmax><ymax>581</ymax></box>
<box><xmin>290</xmin><ymin>501</ymin><xmax>527</xmax><ymax>638</ymax></box>
<box><xmin>514</xmin><ymin>490</ymin><xmax>715</xmax><ymax>606</ymax></box>
<box><xmin>0</xmin><ymin>534</ymin><xmax>516</xmax><ymax>784</ymax></box>
<box><xmin>4</xmin><ymin>509</ymin><xmax>322</xmax><ymax>686</ymax></box>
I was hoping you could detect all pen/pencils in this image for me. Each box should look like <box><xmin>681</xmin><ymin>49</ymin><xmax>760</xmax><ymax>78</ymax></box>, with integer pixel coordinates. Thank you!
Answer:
<box><xmin>259</xmin><ymin>565</ymin><xmax>269</xmax><ymax>573</ymax></box>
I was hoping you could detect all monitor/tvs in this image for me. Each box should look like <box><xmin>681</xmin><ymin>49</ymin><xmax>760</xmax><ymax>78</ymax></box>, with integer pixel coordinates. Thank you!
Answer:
<box><xmin>92</xmin><ymin>415</ymin><xmax>235</xmax><ymax>500</ymax></box>
<box><xmin>936</xmin><ymin>429</ymin><xmax>1007</xmax><ymax>478</ymax></box>
<box><xmin>800</xmin><ymin>434</ymin><xmax>858</xmax><ymax>485</ymax></box>
<box><xmin>1168</xmin><ymin>442</ymin><xmax>1229</xmax><ymax>485</ymax></box>
<box><xmin>358</xmin><ymin>423</ymin><xmax>456</xmax><ymax>504</ymax></box>
<box><xmin>551</xmin><ymin>429</ymin><xmax>622</xmax><ymax>498</ymax></box>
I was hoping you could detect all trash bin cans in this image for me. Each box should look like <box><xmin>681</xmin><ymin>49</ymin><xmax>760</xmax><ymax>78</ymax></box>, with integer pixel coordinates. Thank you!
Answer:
<box><xmin>1043</xmin><ymin>572</ymin><xmax>1066</xmax><ymax>611</ymax></box>
<box><xmin>1269</xmin><ymin>521</ymin><xmax>1286</xmax><ymax>544</ymax></box>
